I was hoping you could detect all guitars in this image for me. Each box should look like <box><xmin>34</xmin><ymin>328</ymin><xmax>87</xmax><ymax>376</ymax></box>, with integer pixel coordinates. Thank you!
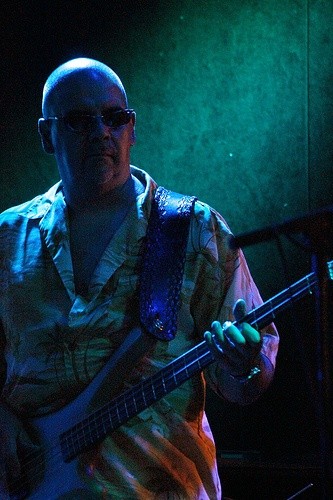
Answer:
<box><xmin>0</xmin><ymin>261</ymin><xmax>333</xmax><ymax>500</ymax></box>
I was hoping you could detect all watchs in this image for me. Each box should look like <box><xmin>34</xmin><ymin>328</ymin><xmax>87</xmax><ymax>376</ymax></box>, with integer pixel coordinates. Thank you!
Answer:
<box><xmin>224</xmin><ymin>363</ymin><xmax>261</xmax><ymax>384</ymax></box>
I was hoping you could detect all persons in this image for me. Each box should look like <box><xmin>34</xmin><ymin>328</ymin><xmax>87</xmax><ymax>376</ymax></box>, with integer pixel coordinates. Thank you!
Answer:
<box><xmin>0</xmin><ymin>58</ymin><xmax>279</xmax><ymax>500</ymax></box>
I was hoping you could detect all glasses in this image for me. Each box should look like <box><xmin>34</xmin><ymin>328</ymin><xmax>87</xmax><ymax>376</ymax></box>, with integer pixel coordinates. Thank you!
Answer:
<box><xmin>45</xmin><ymin>108</ymin><xmax>134</xmax><ymax>129</ymax></box>
